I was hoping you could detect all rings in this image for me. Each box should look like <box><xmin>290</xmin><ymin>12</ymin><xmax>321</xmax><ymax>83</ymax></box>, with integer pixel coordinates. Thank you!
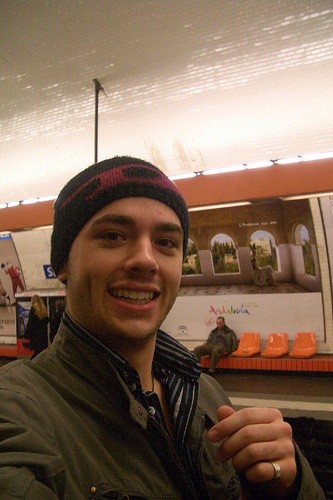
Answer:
<box><xmin>271</xmin><ymin>461</ymin><xmax>281</xmax><ymax>480</ymax></box>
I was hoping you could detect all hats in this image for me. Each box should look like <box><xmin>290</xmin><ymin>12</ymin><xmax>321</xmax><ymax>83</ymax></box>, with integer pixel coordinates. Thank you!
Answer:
<box><xmin>51</xmin><ymin>156</ymin><xmax>188</xmax><ymax>285</ymax></box>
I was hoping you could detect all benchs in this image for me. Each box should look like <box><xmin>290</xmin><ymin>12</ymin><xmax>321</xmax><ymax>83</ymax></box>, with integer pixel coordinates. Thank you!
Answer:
<box><xmin>201</xmin><ymin>332</ymin><xmax>333</xmax><ymax>371</ymax></box>
<box><xmin>0</xmin><ymin>336</ymin><xmax>34</xmax><ymax>359</ymax></box>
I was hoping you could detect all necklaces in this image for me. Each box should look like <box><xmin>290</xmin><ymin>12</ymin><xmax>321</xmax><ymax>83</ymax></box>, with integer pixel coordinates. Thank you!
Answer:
<box><xmin>152</xmin><ymin>373</ymin><xmax>155</xmax><ymax>392</ymax></box>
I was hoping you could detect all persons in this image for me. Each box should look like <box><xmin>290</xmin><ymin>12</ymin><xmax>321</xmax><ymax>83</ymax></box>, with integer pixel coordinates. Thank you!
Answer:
<box><xmin>0</xmin><ymin>156</ymin><xmax>326</xmax><ymax>500</ymax></box>
<box><xmin>0</xmin><ymin>261</ymin><xmax>24</xmax><ymax>306</ymax></box>
<box><xmin>24</xmin><ymin>295</ymin><xmax>50</xmax><ymax>360</ymax></box>
<box><xmin>192</xmin><ymin>316</ymin><xmax>238</xmax><ymax>377</ymax></box>
<box><xmin>50</xmin><ymin>300</ymin><xmax>66</xmax><ymax>344</ymax></box>
